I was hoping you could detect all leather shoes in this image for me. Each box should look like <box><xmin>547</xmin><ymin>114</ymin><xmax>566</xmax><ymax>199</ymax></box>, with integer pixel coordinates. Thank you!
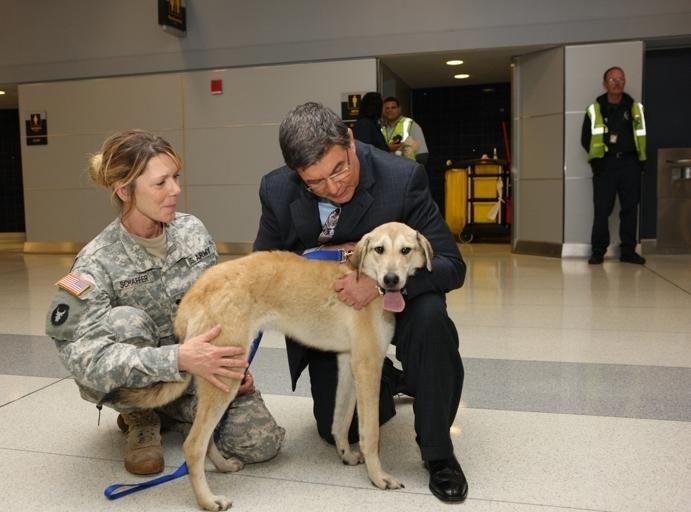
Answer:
<box><xmin>620</xmin><ymin>252</ymin><xmax>645</xmax><ymax>264</ymax></box>
<box><xmin>423</xmin><ymin>454</ymin><xmax>468</xmax><ymax>501</ymax></box>
<box><xmin>588</xmin><ymin>254</ymin><xmax>604</xmax><ymax>264</ymax></box>
<box><xmin>383</xmin><ymin>357</ymin><xmax>415</xmax><ymax>397</ymax></box>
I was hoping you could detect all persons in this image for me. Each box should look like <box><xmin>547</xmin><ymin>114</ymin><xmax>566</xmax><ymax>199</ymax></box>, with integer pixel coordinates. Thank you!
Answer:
<box><xmin>579</xmin><ymin>64</ymin><xmax>650</xmax><ymax>267</ymax></box>
<box><xmin>351</xmin><ymin>92</ymin><xmax>403</xmax><ymax>153</ymax></box>
<box><xmin>42</xmin><ymin>128</ymin><xmax>285</xmax><ymax>476</ymax></box>
<box><xmin>250</xmin><ymin>101</ymin><xmax>468</xmax><ymax>504</ymax></box>
<box><xmin>381</xmin><ymin>96</ymin><xmax>429</xmax><ymax>167</ymax></box>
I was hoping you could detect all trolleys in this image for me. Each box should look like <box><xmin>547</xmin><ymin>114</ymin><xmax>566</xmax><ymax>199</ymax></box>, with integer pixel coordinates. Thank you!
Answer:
<box><xmin>460</xmin><ymin>159</ymin><xmax>513</xmax><ymax>243</ymax></box>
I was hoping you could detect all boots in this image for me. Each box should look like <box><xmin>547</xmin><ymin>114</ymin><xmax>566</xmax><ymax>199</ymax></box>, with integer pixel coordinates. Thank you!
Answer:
<box><xmin>118</xmin><ymin>407</ymin><xmax>173</xmax><ymax>475</ymax></box>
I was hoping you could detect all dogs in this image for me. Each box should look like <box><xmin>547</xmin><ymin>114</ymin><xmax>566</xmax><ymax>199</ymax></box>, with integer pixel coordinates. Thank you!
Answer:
<box><xmin>97</xmin><ymin>220</ymin><xmax>435</xmax><ymax>512</ymax></box>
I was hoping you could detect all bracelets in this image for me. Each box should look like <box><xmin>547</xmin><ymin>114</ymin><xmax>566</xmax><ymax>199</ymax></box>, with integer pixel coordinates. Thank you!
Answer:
<box><xmin>373</xmin><ymin>283</ymin><xmax>385</xmax><ymax>296</ymax></box>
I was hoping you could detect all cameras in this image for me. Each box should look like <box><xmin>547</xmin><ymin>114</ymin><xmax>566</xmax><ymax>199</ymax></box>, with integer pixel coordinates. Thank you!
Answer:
<box><xmin>392</xmin><ymin>134</ymin><xmax>403</xmax><ymax>144</ymax></box>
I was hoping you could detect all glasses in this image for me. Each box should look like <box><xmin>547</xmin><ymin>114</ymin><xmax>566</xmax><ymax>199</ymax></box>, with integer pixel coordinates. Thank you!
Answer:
<box><xmin>305</xmin><ymin>148</ymin><xmax>351</xmax><ymax>193</ymax></box>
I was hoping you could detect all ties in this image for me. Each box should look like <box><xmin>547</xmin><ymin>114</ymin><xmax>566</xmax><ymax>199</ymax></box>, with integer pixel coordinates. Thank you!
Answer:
<box><xmin>318</xmin><ymin>209</ymin><xmax>341</xmax><ymax>244</ymax></box>
